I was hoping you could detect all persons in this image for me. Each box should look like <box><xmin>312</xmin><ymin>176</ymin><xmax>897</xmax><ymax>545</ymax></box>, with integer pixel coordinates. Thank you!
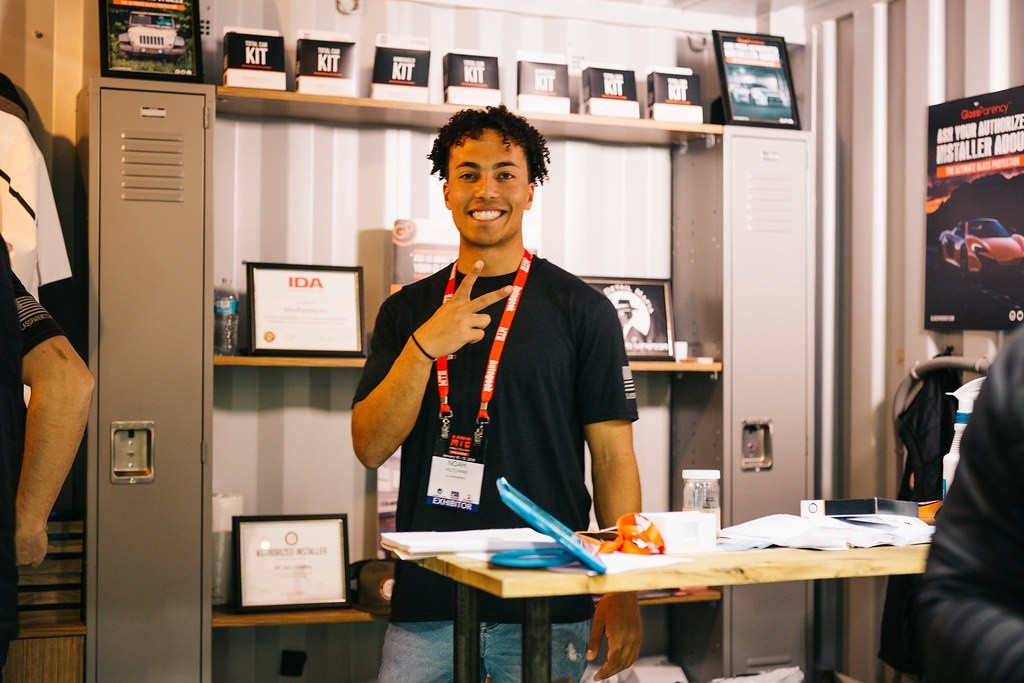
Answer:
<box><xmin>349</xmin><ymin>106</ymin><xmax>644</xmax><ymax>683</ymax></box>
<box><xmin>0</xmin><ymin>234</ymin><xmax>100</xmax><ymax>682</ymax></box>
<box><xmin>904</xmin><ymin>320</ymin><xmax>1024</xmax><ymax>683</ymax></box>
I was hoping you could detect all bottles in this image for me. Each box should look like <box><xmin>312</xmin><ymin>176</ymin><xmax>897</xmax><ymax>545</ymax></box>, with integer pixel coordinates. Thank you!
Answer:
<box><xmin>681</xmin><ymin>469</ymin><xmax>722</xmax><ymax>541</ymax></box>
<box><xmin>214</xmin><ymin>277</ymin><xmax>239</xmax><ymax>355</ymax></box>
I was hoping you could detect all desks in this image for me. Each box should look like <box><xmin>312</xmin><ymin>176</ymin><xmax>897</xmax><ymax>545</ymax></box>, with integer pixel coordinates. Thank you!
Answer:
<box><xmin>380</xmin><ymin>531</ymin><xmax>931</xmax><ymax>682</ymax></box>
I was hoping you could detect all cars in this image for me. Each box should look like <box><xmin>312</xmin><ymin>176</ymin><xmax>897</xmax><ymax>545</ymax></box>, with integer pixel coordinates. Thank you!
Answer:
<box><xmin>733</xmin><ymin>82</ymin><xmax>782</xmax><ymax>108</ymax></box>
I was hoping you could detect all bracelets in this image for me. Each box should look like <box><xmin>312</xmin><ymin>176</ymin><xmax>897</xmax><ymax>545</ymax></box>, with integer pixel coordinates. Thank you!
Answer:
<box><xmin>409</xmin><ymin>333</ymin><xmax>441</xmax><ymax>363</ymax></box>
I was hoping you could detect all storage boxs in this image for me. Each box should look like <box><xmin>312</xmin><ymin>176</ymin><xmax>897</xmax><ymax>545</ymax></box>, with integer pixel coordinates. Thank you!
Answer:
<box><xmin>647</xmin><ymin>66</ymin><xmax>703</xmax><ymax>124</ymax></box>
<box><xmin>223</xmin><ymin>26</ymin><xmax>287</xmax><ymax>91</ymax></box>
<box><xmin>800</xmin><ymin>497</ymin><xmax>919</xmax><ymax>519</ymax></box>
<box><xmin>517</xmin><ymin>60</ymin><xmax>570</xmax><ymax>114</ymax></box>
<box><xmin>294</xmin><ymin>30</ymin><xmax>359</xmax><ymax>98</ymax></box>
<box><xmin>582</xmin><ymin>62</ymin><xmax>641</xmax><ymax>119</ymax></box>
<box><xmin>369</xmin><ymin>44</ymin><xmax>432</xmax><ymax>104</ymax></box>
<box><xmin>442</xmin><ymin>52</ymin><xmax>503</xmax><ymax>108</ymax></box>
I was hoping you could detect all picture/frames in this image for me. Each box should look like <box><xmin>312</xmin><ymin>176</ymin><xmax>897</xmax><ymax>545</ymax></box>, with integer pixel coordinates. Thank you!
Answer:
<box><xmin>711</xmin><ymin>28</ymin><xmax>802</xmax><ymax>131</ymax></box>
<box><xmin>575</xmin><ymin>277</ymin><xmax>676</xmax><ymax>361</ymax></box>
<box><xmin>98</xmin><ymin>0</ymin><xmax>206</xmax><ymax>84</ymax></box>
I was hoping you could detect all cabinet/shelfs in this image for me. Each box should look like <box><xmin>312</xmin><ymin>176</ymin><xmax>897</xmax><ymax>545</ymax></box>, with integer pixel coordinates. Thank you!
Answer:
<box><xmin>86</xmin><ymin>76</ymin><xmax>216</xmax><ymax>683</ymax></box>
<box><xmin>670</xmin><ymin>123</ymin><xmax>817</xmax><ymax>683</ymax></box>
<box><xmin>210</xmin><ymin>85</ymin><xmax>722</xmax><ymax>629</ymax></box>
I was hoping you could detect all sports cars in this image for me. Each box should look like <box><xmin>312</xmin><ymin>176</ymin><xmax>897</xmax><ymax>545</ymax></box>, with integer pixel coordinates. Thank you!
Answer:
<box><xmin>939</xmin><ymin>218</ymin><xmax>1024</xmax><ymax>281</ymax></box>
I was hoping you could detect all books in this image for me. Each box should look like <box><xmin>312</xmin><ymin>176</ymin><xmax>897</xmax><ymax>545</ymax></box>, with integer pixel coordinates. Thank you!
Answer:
<box><xmin>381</xmin><ymin>527</ymin><xmax>556</xmax><ymax>555</ymax></box>
